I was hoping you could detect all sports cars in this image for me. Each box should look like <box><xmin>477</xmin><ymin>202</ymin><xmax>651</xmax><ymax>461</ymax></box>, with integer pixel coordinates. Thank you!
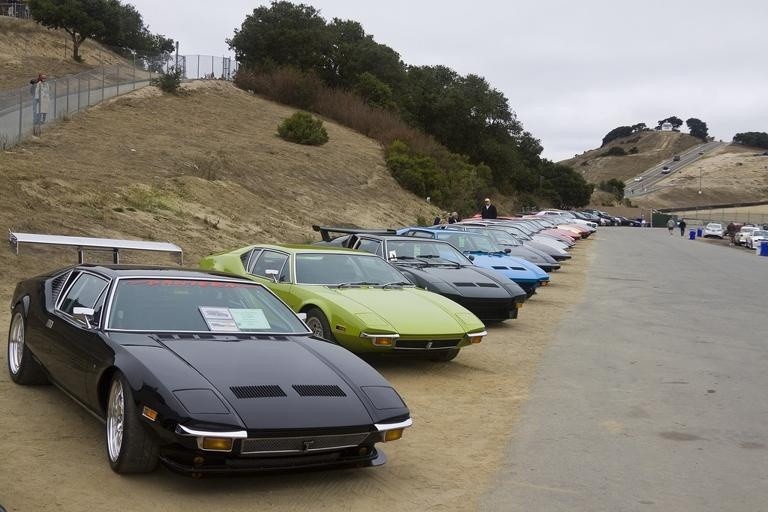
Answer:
<box><xmin>198</xmin><ymin>242</ymin><xmax>489</xmax><ymax>363</ymax></box>
<box><xmin>393</xmin><ymin>208</ymin><xmax>646</xmax><ymax>300</ymax></box>
<box><xmin>311</xmin><ymin>223</ymin><xmax>527</xmax><ymax>325</ymax></box>
<box><xmin>4</xmin><ymin>261</ymin><xmax>415</xmax><ymax>481</ymax></box>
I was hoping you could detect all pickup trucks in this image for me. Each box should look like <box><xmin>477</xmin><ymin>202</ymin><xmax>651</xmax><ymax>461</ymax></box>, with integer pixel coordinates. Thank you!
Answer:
<box><xmin>662</xmin><ymin>166</ymin><xmax>671</xmax><ymax>173</ymax></box>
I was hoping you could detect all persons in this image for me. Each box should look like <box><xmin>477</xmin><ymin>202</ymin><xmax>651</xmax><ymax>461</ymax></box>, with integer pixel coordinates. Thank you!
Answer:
<box><xmin>667</xmin><ymin>217</ymin><xmax>687</xmax><ymax>236</ymax></box>
<box><xmin>727</xmin><ymin>221</ymin><xmax>736</xmax><ymax>246</ymax></box>
<box><xmin>433</xmin><ymin>210</ymin><xmax>461</xmax><ymax>225</ymax></box>
<box><xmin>480</xmin><ymin>197</ymin><xmax>498</xmax><ymax>220</ymax></box>
<box><xmin>30</xmin><ymin>74</ymin><xmax>51</xmax><ymax>126</ymax></box>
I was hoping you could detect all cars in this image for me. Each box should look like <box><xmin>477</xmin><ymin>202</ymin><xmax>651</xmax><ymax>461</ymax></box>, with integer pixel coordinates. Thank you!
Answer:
<box><xmin>635</xmin><ymin>177</ymin><xmax>642</xmax><ymax>182</ymax></box>
<box><xmin>703</xmin><ymin>222</ymin><xmax>768</xmax><ymax>249</ymax></box>
